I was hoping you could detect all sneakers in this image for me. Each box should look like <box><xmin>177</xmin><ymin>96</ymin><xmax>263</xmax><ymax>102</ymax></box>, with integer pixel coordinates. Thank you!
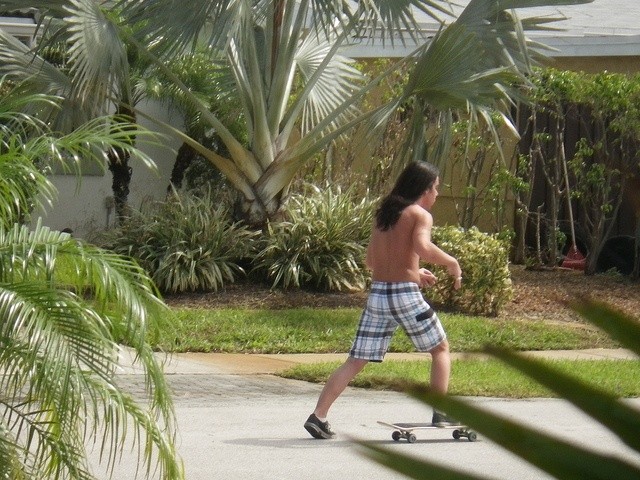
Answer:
<box><xmin>432</xmin><ymin>413</ymin><xmax>462</xmax><ymax>427</ymax></box>
<box><xmin>304</xmin><ymin>414</ymin><xmax>336</xmax><ymax>439</ymax></box>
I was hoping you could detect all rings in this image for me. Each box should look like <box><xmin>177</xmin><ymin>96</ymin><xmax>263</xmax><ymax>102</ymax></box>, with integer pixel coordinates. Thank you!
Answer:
<box><xmin>455</xmin><ymin>278</ymin><xmax>462</xmax><ymax>283</ymax></box>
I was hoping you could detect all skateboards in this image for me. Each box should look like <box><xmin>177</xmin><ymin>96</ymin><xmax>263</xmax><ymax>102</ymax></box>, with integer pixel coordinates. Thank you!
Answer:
<box><xmin>378</xmin><ymin>421</ymin><xmax>477</xmax><ymax>442</ymax></box>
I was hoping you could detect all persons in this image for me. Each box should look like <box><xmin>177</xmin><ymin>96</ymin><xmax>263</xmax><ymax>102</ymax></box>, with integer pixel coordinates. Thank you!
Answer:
<box><xmin>304</xmin><ymin>159</ymin><xmax>462</xmax><ymax>439</ymax></box>
